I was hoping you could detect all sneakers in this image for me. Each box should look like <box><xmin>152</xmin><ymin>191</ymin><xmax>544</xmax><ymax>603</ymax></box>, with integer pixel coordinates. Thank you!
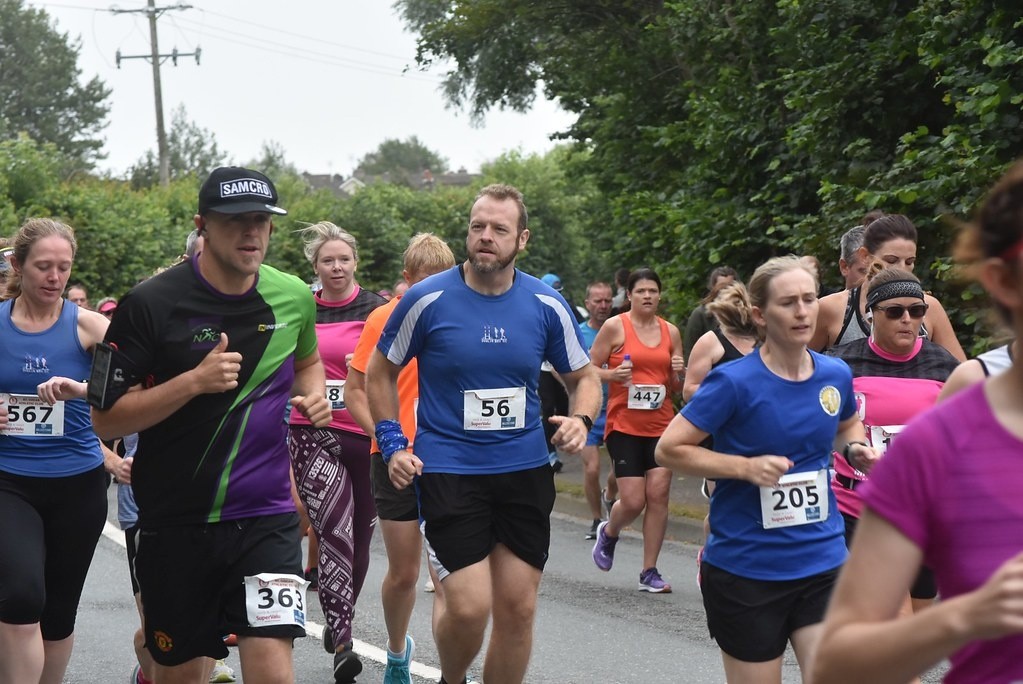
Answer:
<box><xmin>210</xmin><ymin>657</ymin><xmax>236</xmax><ymax>683</ymax></box>
<box><xmin>322</xmin><ymin>625</ymin><xmax>337</xmax><ymax>654</ymax></box>
<box><xmin>383</xmin><ymin>635</ymin><xmax>416</xmax><ymax>684</ymax></box>
<box><xmin>637</xmin><ymin>567</ymin><xmax>671</xmax><ymax>592</ymax></box>
<box><xmin>592</xmin><ymin>521</ymin><xmax>619</xmax><ymax>571</ymax></box>
<box><xmin>333</xmin><ymin>651</ymin><xmax>363</xmax><ymax>682</ymax></box>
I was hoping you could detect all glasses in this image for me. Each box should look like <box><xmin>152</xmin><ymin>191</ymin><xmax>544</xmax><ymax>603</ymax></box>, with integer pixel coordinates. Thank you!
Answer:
<box><xmin>875</xmin><ymin>302</ymin><xmax>929</xmax><ymax>320</ymax></box>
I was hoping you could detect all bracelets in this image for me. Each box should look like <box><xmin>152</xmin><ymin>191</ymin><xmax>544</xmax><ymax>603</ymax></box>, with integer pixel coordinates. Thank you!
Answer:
<box><xmin>843</xmin><ymin>440</ymin><xmax>868</xmax><ymax>468</ymax></box>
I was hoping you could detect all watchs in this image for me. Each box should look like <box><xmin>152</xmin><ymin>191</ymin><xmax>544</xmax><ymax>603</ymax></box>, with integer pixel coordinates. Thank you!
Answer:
<box><xmin>574</xmin><ymin>413</ymin><xmax>593</xmax><ymax>431</ymax></box>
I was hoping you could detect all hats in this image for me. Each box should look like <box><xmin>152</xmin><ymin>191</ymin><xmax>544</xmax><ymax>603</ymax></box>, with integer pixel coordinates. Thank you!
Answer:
<box><xmin>199</xmin><ymin>166</ymin><xmax>288</xmax><ymax>218</ymax></box>
<box><xmin>542</xmin><ymin>274</ymin><xmax>564</xmax><ymax>292</ymax></box>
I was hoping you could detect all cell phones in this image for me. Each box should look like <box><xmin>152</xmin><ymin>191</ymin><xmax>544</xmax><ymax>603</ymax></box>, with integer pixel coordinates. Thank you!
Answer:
<box><xmin>86</xmin><ymin>342</ymin><xmax>113</xmax><ymax>408</ymax></box>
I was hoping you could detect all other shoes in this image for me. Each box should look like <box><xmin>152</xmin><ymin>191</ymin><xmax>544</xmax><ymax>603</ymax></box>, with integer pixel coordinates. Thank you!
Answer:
<box><xmin>424</xmin><ymin>573</ymin><xmax>435</xmax><ymax>591</ymax></box>
<box><xmin>585</xmin><ymin>518</ymin><xmax>602</xmax><ymax>540</ymax></box>
<box><xmin>549</xmin><ymin>452</ymin><xmax>563</xmax><ymax>472</ymax></box>
<box><xmin>304</xmin><ymin>566</ymin><xmax>320</xmax><ymax>592</ymax></box>
<box><xmin>602</xmin><ymin>487</ymin><xmax>617</xmax><ymax>520</ymax></box>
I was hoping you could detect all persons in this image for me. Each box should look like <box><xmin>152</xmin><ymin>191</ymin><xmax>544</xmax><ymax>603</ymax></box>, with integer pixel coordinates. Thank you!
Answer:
<box><xmin>0</xmin><ymin>167</ymin><xmax>1022</xmax><ymax>684</ymax></box>
